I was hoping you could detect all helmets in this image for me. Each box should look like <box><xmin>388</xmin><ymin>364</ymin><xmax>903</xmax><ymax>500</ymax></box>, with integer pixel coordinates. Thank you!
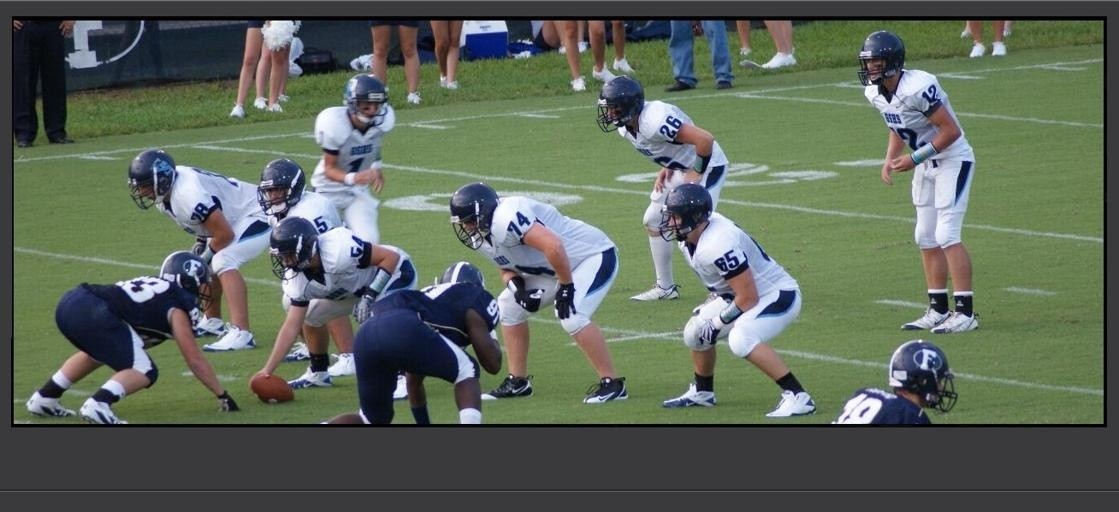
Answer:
<box><xmin>344</xmin><ymin>74</ymin><xmax>388</xmax><ymax>126</ymax></box>
<box><xmin>659</xmin><ymin>184</ymin><xmax>712</xmax><ymax>241</ymax></box>
<box><xmin>257</xmin><ymin>158</ymin><xmax>318</xmax><ymax>280</ymax></box>
<box><xmin>441</xmin><ymin>261</ymin><xmax>483</xmax><ymax>288</ymax></box>
<box><xmin>597</xmin><ymin>75</ymin><xmax>644</xmax><ymax>132</ymax></box>
<box><xmin>128</xmin><ymin>150</ymin><xmax>176</xmax><ymax>208</ymax></box>
<box><xmin>451</xmin><ymin>182</ymin><xmax>499</xmax><ymax>249</ymax></box>
<box><xmin>858</xmin><ymin>31</ymin><xmax>905</xmax><ymax>85</ymax></box>
<box><xmin>160</xmin><ymin>251</ymin><xmax>214</xmax><ymax>311</ymax></box>
<box><xmin>888</xmin><ymin>340</ymin><xmax>957</xmax><ymax>412</ymax></box>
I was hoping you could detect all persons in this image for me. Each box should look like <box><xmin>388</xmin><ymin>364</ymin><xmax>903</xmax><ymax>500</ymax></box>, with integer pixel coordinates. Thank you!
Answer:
<box><xmin>832</xmin><ymin>339</ymin><xmax>958</xmax><ymax>427</ymax></box>
<box><xmin>13</xmin><ymin>18</ymin><xmax>75</xmax><ymax>148</ymax></box>
<box><xmin>857</xmin><ymin>29</ymin><xmax>980</xmax><ymax>333</ymax></box>
<box><xmin>959</xmin><ymin>17</ymin><xmax>1013</xmax><ymax>58</ymax></box>
<box><xmin>446</xmin><ymin>182</ymin><xmax>631</xmax><ymax>406</ymax></box>
<box><xmin>529</xmin><ymin>18</ymin><xmax>636</xmax><ymax>92</ymax></box>
<box><xmin>230</xmin><ymin>20</ymin><xmax>304</xmax><ymax>116</ymax></box>
<box><xmin>595</xmin><ymin>75</ymin><xmax>729</xmax><ymax>303</ymax></box>
<box><xmin>736</xmin><ymin>19</ymin><xmax>799</xmax><ymax>69</ymax></box>
<box><xmin>430</xmin><ymin>21</ymin><xmax>464</xmax><ymax>89</ymax></box>
<box><xmin>24</xmin><ymin>149</ymin><xmax>504</xmax><ymax>428</ymax></box>
<box><xmin>658</xmin><ymin>182</ymin><xmax>817</xmax><ymax>418</ymax></box>
<box><xmin>308</xmin><ymin>73</ymin><xmax>396</xmax><ymax>246</ymax></box>
<box><xmin>663</xmin><ymin>18</ymin><xmax>735</xmax><ymax>92</ymax></box>
<box><xmin>367</xmin><ymin>17</ymin><xmax>424</xmax><ymax>106</ymax></box>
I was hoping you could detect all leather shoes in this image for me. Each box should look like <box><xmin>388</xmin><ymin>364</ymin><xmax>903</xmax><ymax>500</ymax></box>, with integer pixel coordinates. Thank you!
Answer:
<box><xmin>18</xmin><ymin>135</ymin><xmax>73</xmax><ymax>147</ymax></box>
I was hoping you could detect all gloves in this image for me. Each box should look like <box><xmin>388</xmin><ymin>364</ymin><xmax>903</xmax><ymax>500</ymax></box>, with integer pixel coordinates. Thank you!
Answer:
<box><xmin>354</xmin><ymin>293</ymin><xmax>374</xmax><ymax>323</ymax></box>
<box><xmin>218</xmin><ymin>391</ymin><xmax>239</xmax><ymax>412</ymax></box>
<box><xmin>699</xmin><ymin>314</ymin><xmax>727</xmax><ymax>345</ymax></box>
<box><xmin>515</xmin><ymin>288</ymin><xmax>544</xmax><ymax>311</ymax></box>
<box><xmin>554</xmin><ymin>283</ymin><xmax>576</xmax><ymax>318</ymax></box>
<box><xmin>192</xmin><ymin>237</ymin><xmax>215</xmax><ymax>262</ymax></box>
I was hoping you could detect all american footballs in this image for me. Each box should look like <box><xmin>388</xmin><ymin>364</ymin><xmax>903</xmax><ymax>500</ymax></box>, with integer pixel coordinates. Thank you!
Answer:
<box><xmin>249</xmin><ymin>373</ymin><xmax>296</xmax><ymax>403</ymax></box>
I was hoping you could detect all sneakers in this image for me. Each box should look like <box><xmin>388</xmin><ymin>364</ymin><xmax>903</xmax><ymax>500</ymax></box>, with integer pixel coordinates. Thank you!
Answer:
<box><xmin>284</xmin><ymin>342</ymin><xmax>357</xmax><ymax>389</ymax></box>
<box><xmin>766</xmin><ymin>390</ymin><xmax>816</xmax><ymax>417</ymax></box>
<box><xmin>230</xmin><ymin>94</ymin><xmax>288</xmax><ymax>117</ymax></box>
<box><xmin>666</xmin><ymin>81</ymin><xmax>731</xmax><ymax>91</ymax></box>
<box><xmin>582</xmin><ymin>377</ymin><xmax>628</xmax><ymax>403</ymax></box>
<box><xmin>630</xmin><ymin>284</ymin><xmax>681</xmax><ymax>300</ymax></box>
<box><xmin>481</xmin><ymin>373</ymin><xmax>533</xmax><ymax>399</ymax></box>
<box><xmin>740</xmin><ymin>49</ymin><xmax>797</xmax><ymax>68</ymax></box>
<box><xmin>27</xmin><ymin>392</ymin><xmax>76</xmax><ymax>417</ymax></box>
<box><xmin>80</xmin><ymin>397</ymin><xmax>127</xmax><ymax>426</ymax></box>
<box><xmin>409</xmin><ymin>74</ymin><xmax>459</xmax><ymax>103</ymax></box>
<box><xmin>664</xmin><ymin>383</ymin><xmax>716</xmax><ymax>408</ymax></box>
<box><xmin>557</xmin><ymin>42</ymin><xmax>634</xmax><ymax>90</ymax></box>
<box><xmin>961</xmin><ymin>23</ymin><xmax>1012</xmax><ymax>58</ymax></box>
<box><xmin>392</xmin><ymin>373</ymin><xmax>408</xmax><ymax>399</ymax></box>
<box><xmin>901</xmin><ymin>306</ymin><xmax>979</xmax><ymax>333</ymax></box>
<box><xmin>195</xmin><ymin>314</ymin><xmax>256</xmax><ymax>350</ymax></box>
